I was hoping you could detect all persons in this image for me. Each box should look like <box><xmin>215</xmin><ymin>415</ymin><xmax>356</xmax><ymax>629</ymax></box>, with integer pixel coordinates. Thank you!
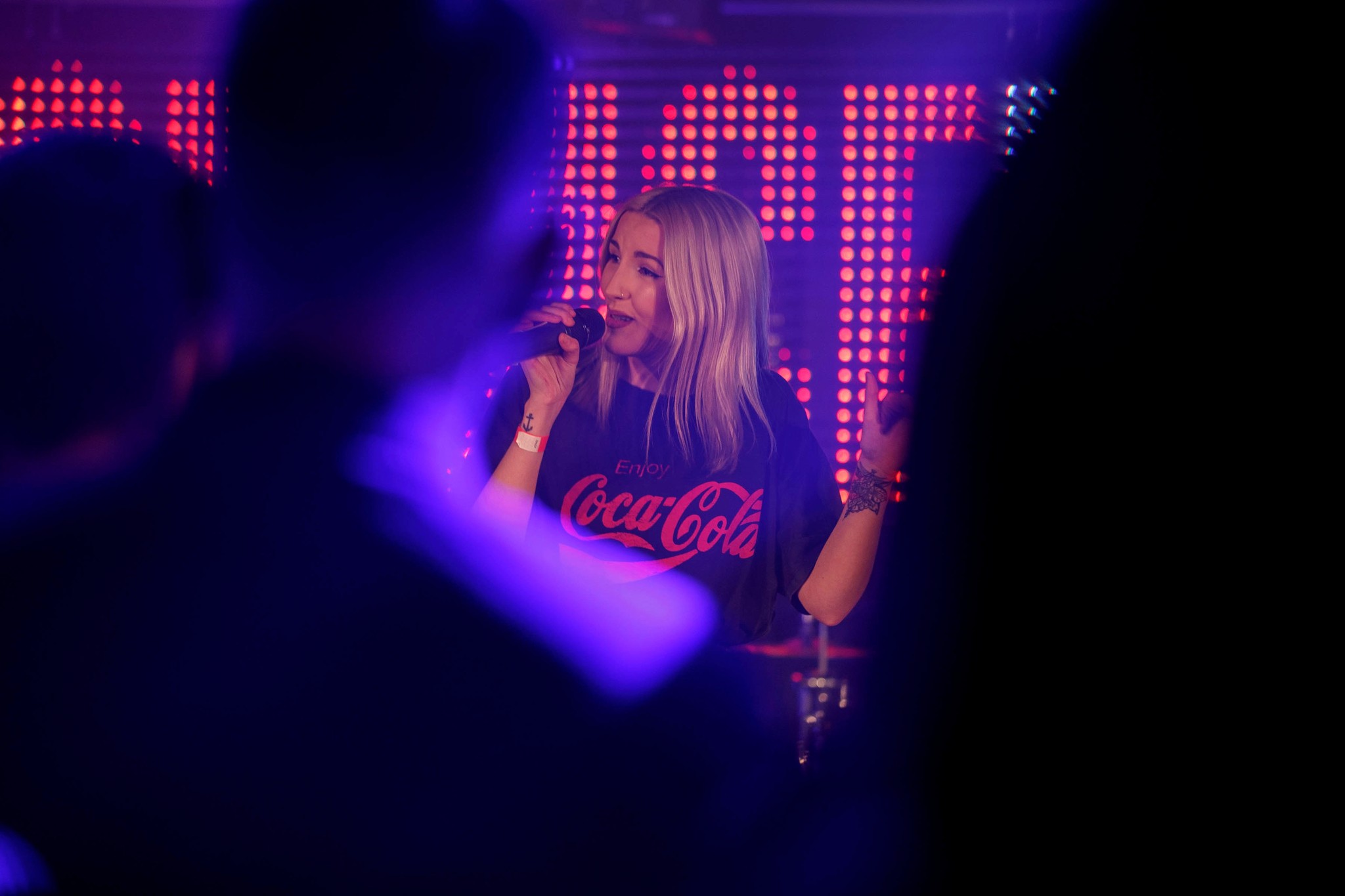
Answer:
<box><xmin>468</xmin><ymin>182</ymin><xmax>915</xmax><ymax>653</ymax></box>
<box><xmin>0</xmin><ymin>0</ymin><xmax>797</xmax><ymax>896</ymax></box>
<box><xmin>0</xmin><ymin>126</ymin><xmax>226</xmax><ymax>896</ymax></box>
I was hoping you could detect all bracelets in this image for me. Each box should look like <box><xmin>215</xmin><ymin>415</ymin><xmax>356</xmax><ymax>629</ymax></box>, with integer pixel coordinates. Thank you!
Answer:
<box><xmin>511</xmin><ymin>423</ymin><xmax>550</xmax><ymax>453</ymax></box>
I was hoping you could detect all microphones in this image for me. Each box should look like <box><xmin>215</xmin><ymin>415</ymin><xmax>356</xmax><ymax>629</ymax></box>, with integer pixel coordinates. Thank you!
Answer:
<box><xmin>505</xmin><ymin>305</ymin><xmax>607</xmax><ymax>364</ymax></box>
